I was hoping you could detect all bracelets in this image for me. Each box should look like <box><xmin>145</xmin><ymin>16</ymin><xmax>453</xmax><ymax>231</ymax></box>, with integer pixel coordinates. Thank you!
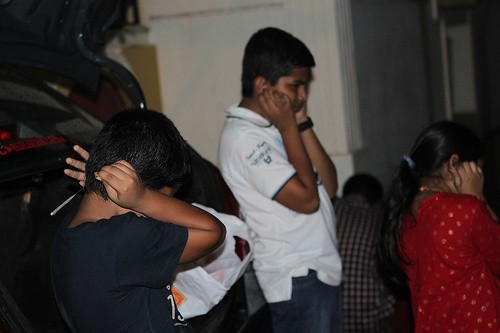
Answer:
<box><xmin>296</xmin><ymin>116</ymin><xmax>313</xmax><ymax>132</ymax></box>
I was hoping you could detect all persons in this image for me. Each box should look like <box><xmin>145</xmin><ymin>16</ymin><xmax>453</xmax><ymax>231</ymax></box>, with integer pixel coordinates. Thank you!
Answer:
<box><xmin>50</xmin><ymin>28</ymin><xmax>500</xmax><ymax>333</ymax></box>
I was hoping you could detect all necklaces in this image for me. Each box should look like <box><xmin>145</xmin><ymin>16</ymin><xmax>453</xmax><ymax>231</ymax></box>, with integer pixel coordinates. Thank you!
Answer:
<box><xmin>418</xmin><ymin>184</ymin><xmax>444</xmax><ymax>194</ymax></box>
